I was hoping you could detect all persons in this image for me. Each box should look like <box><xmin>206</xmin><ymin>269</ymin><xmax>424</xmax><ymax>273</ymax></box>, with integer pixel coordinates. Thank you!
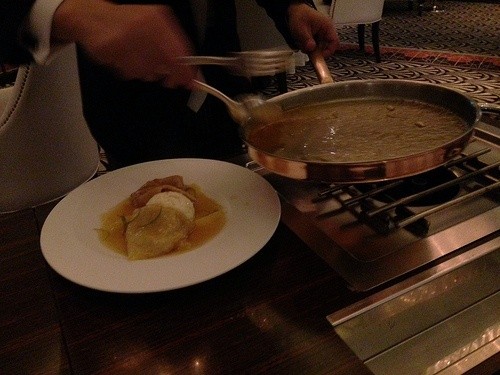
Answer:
<box><xmin>0</xmin><ymin>0</ymin><xmax>339</xmax><ymax>170</ymax></box>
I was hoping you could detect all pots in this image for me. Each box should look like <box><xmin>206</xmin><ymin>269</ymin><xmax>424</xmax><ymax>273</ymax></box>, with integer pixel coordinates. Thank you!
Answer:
<box><xmin>175</xmin><ymin>79</ymin><xmax>483</xmax><ymax>185</ymax></box>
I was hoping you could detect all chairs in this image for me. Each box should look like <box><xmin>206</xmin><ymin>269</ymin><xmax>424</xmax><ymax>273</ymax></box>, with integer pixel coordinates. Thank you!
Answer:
<box><xmin>320</xmin><ymin>1</ymin><xmax>385</xmax><ymax>70</ymax></box>
<box><xmin>0</xmin><ymin>44</ymin><xmax>102</xmax><ymax>222</ymax></box>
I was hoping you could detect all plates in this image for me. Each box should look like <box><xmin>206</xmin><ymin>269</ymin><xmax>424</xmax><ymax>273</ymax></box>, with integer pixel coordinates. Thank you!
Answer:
<box><xmin>40</xmin><ymin>158</ymin><xmax>281</xmax><ymax>294</ymax></box>
